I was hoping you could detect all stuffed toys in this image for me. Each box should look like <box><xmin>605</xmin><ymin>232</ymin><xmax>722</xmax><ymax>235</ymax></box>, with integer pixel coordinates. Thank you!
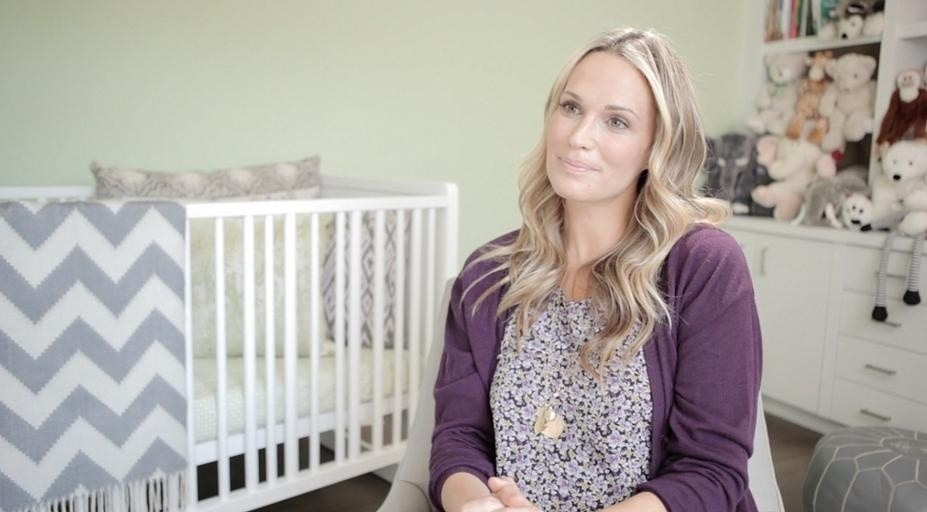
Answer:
<box><xmin>700</xmin><ymin>50</ymin><xmax>905</xmax><ymax>232</ymax></box>
<box><xmin>872</xmin><ymin>67</ymin><xmax>927</xmax><ymax>324</ymax></box>
<box><xmin>698</xmin><ymin>0</ymin><xmax>927</xmax><ymax>322</ymax></box>
<box><xmin>819</xmin><ymin>1</ymin><xmax>885</xmax><ymax>40</ymax></box>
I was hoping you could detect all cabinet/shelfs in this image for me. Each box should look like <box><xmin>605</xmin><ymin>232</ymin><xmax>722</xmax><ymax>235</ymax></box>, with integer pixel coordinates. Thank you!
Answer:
<box><xmin>704</xmin><ymin>1</ymin><xmax>927</xmax><ymax>440</ymax></box>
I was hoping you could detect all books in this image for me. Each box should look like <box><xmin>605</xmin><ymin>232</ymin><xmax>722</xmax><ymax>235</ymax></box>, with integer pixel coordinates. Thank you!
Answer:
<box><xmin>768</xmin><ymin>1</ymin><xmax>819</xmax><ymax>39</ymax></box>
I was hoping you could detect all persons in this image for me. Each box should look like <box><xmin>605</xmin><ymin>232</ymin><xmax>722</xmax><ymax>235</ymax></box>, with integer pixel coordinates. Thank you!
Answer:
<box><xmin>427</xmin><ymin>26</ymin><xmax>764</xmax><ymax>512</ymax></box>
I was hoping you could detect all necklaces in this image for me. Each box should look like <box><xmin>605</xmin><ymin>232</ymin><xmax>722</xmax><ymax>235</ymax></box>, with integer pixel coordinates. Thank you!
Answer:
<box><xmin>534</xmin><ymin>288</ymin><xmax>592</xmax><ymax>438</ymax></box>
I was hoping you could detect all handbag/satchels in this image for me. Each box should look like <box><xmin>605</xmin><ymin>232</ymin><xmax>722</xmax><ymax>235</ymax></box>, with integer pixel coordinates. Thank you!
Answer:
<box><xmin>704</xmin><ymin>132</ymin><xmax>758</xmax><ymax>202</ymax></box>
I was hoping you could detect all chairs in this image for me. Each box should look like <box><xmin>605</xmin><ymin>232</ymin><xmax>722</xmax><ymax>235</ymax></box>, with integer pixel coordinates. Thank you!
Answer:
<box><xmin>365</xmin><ymin>276</ymin><xmax>788</xmax><ymax>512</ymax></box>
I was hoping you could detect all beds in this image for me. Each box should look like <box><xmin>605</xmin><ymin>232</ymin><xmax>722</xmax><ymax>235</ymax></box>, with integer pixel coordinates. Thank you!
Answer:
<box><xmin>0</xmin><ymin>182</ymin><xmax>460</xmax><ymax>510</ymax></box>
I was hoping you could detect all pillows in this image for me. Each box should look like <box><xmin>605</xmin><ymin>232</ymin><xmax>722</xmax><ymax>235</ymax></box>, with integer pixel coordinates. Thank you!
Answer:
<box><xmin>93</xmin><ymin>157</ymin><xmax>328</xmax><ymax>358</ymax></box>
